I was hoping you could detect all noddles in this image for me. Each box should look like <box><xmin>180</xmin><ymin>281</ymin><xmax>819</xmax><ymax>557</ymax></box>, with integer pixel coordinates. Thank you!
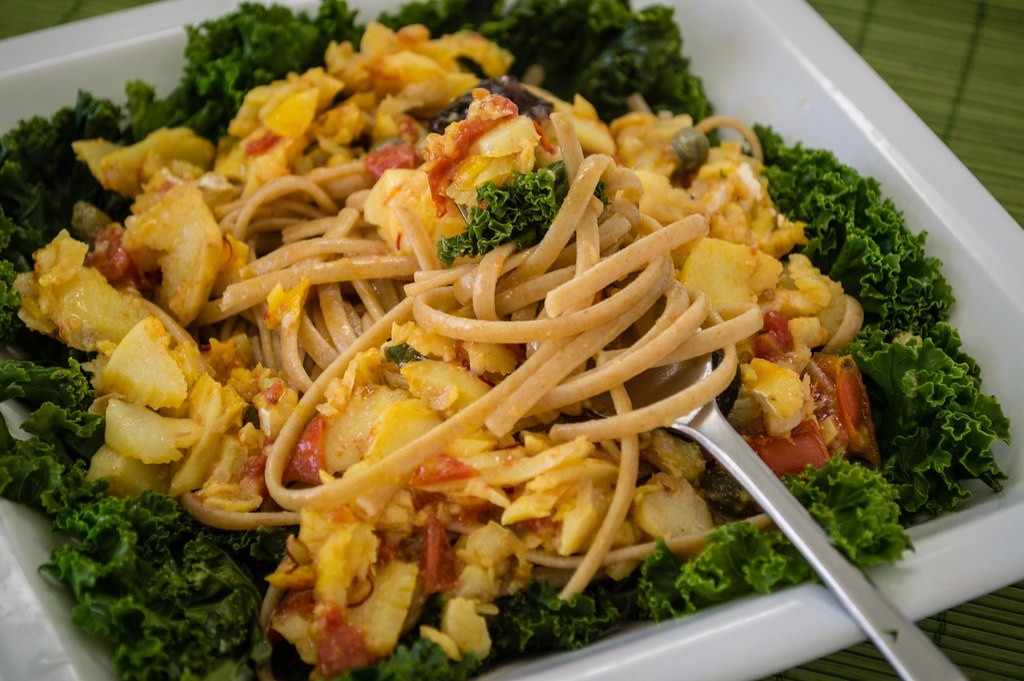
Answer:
<box><xmin>221</xmin><ymin>113</ymin><xmax>774</xmax><ymax>603</ymax></box>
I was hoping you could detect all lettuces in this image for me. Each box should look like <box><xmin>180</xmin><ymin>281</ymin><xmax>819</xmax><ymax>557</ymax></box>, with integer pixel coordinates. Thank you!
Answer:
<box><xmin>1</xmin><ymin>0</ymin><xmax>1011</xmax><ymax>681</ymax></box>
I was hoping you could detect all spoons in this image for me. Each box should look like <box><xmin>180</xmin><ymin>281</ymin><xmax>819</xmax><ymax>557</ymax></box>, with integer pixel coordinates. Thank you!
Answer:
<box><xmin>583</xmin><ymin>290</ymin><xmax>967</xmax><ymax>681</ymax></box>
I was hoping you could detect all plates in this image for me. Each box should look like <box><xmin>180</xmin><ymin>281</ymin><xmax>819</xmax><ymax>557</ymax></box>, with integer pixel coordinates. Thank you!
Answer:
<box><xmin>0</xmin><ymin>0</ymin><xmax>1024</xmax><ymax>681</ymax></box>
